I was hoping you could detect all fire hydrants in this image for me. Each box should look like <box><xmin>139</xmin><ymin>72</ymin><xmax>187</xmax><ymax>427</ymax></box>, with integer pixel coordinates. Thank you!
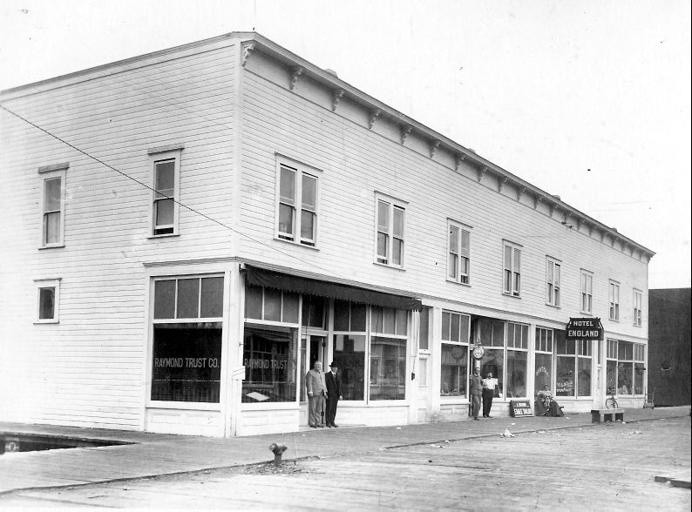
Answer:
<box><xmin>269</xmin><ymin>442</ymin><xmax>287</xmax><ymax>466</ymax></box>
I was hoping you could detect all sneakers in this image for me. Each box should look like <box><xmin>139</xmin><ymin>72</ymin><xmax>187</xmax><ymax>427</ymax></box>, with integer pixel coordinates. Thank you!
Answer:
<box><xmin>310</xmin><ymin>423</ymin><xmax>337</xmax><ymax>428</ymax></box>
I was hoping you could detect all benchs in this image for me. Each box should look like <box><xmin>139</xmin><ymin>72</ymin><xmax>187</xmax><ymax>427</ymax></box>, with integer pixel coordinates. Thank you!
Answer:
<box><xmin>591</xmin><ymin>408</ymin><xmax>624</xmax><ymax>423</ymax></box>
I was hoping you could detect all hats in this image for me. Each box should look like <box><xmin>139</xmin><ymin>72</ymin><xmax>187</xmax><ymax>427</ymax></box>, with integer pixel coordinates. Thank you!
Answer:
<box><xmin>329</xmin><ymin>362</ymin><xmax>338</xmax><ymax>366</ymax></box>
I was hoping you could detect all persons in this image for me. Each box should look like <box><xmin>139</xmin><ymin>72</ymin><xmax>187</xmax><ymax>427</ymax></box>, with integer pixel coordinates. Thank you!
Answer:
<box><xmin>323</xmin><ymin>360</ymin><xmax>343</xmax><ymax>428</ymax></box>
<box><xmin>304</xmin><ymin>360</ymin><xmax>328</xmax><ymax>428</ymax></box>
<box><xmin>481</xmin><ymin>370</ymin><xmax>496</xmax><ymax>419</ymax></box>
<box><xmin>468</xmin><ymin>367</ymin><xmax>483</xmax><ymax>421</ymax></box>
